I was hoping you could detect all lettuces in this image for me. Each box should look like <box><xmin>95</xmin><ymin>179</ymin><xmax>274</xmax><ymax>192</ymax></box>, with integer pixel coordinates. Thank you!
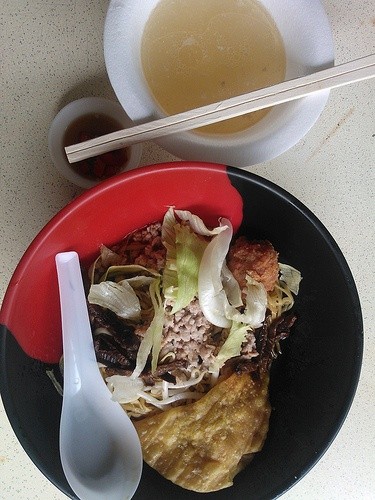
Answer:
<box><xmin>87</xmin><ymin>205</ymin><xmax>300</xmax><ymax>403</ymax></box>
